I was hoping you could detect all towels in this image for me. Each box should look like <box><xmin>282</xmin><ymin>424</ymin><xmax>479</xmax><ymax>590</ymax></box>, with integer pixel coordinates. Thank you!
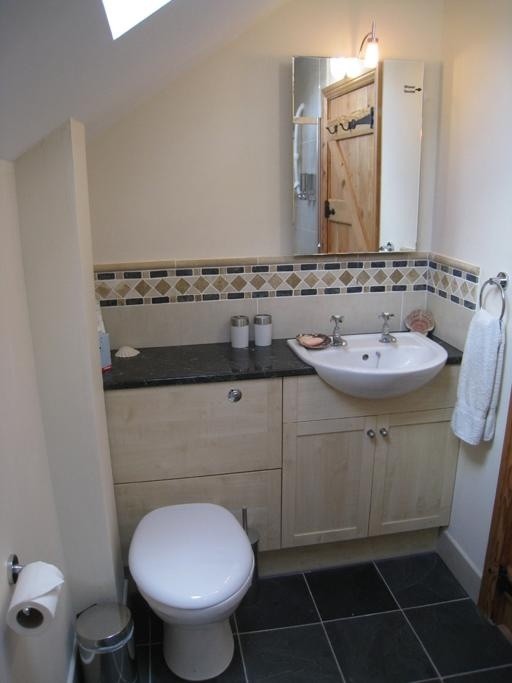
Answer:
<box><xmin>452</xmin><ymin>309</ymin><xmax>505</xmax><ymax>444</ymax></box>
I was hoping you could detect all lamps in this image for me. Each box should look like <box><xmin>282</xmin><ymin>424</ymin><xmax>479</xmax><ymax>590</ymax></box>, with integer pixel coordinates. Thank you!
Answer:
<box><xmin>359</xmin><ymin>22</ymin><xmax>380</xmax><ymax>68</ymax></box>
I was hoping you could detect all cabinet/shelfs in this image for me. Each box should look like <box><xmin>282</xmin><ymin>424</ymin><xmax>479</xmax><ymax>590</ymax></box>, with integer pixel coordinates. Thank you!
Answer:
<box><xmin>282</xmin><ymin>364</ymin><xmax>461</xmax><ymax>549</ymax></box>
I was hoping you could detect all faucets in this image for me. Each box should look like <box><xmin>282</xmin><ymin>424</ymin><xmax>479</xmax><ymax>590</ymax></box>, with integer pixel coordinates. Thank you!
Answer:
<box><xmin>329</xmin><ymin>314</ymin><xmax>347</xmax><ymax>347</ymax></box>
<box><xmin>378</xmin><ymin>311</ymin><xmax>397</xmax><ymax>343</ymax></box>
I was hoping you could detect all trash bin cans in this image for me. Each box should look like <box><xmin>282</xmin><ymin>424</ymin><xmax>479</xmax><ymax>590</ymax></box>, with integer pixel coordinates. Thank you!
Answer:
<box><xmin>76</xmin><ymin>603</ymin><xmax>140</xmax><ymax>683</ymax></box>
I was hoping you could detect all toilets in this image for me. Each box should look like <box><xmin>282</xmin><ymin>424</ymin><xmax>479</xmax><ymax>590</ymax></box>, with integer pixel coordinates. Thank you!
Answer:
<box><xmin>128</xmin><ymin>502</ymin><xmax>254</xmax><ymax>681</ymax></box>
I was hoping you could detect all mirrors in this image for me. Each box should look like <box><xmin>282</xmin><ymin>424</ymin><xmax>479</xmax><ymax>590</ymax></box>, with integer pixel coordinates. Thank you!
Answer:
<box><xmin>292</xmin><ymin>56</ymin><xmax>425</xmax><ymax>257</ymax></box>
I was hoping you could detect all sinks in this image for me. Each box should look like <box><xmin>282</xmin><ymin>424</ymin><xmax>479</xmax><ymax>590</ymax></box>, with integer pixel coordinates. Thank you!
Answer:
<box><xmin>287</xmin><ymin>332</ymin><xmax>448</xmax><ymax>400</ymax></box>
